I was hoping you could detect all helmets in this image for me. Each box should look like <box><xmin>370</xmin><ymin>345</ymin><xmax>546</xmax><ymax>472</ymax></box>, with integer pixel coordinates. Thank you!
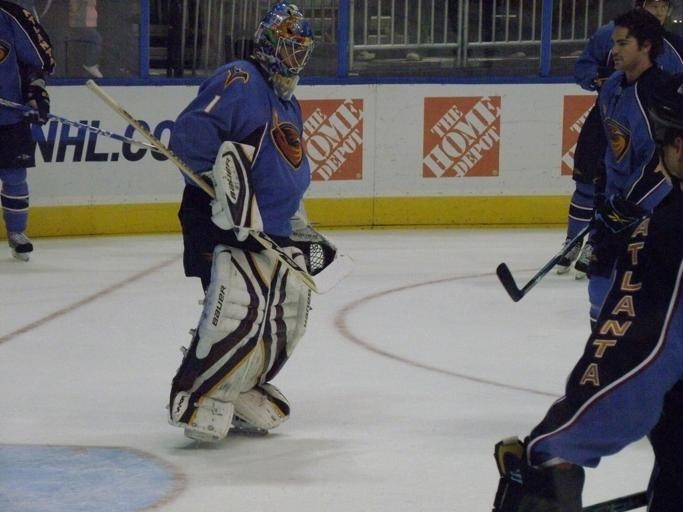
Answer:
<box><xmin>633</xmin><ymin>71</ymin><xmax>682</xmax><ymax>147</ymax></box>
<box><xmin>252</xmin><ymin>1</ymin><xmax>316</xmax><ymax>78</ymax></box>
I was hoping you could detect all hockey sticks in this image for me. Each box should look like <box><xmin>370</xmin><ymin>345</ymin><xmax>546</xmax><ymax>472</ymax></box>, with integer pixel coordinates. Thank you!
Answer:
<box><xmin>86</xmin><ymin>80</ymin><xmax>356</xmax><ymax>294</ymax></box>
<box><xmin>496</xmin><ymin>221</ymin><xmax>594</xmax><ymax>302</ymax></box>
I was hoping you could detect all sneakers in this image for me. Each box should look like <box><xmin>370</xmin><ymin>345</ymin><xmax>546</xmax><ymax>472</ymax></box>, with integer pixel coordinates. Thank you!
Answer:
<box><xmin>7</xmin><ymin>231</ymin><xmax>34</xmax><ymax>253</ymax></box>
<box><xmin>575</xmin><ymin>241</ymin><xmax>594</xmax><ymax>270</ymax></box>
<box><xmin>555</xmin><ymin>236</ymin><xmax>584</xmax><ymax>267</ymax></box>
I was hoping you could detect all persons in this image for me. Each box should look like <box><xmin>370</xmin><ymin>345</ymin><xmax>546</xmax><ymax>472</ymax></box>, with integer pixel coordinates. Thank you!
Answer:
<box><xmin>167</xmin><ymin>2</ymin><xmax>337</xmax><ymax>437</ymax></box>
<box><xmin>560</xmin><ymin>2</ymin><xmax>683</xmax><ymax>277</ymax></box>
<box><xmin>6</xmin><ymin>1</ymin><xmax>682</xmax><ymax>81</ymax></box>
<box><xmin>493</xmin><ymin>72</ymin><xmax>683</xmax><ymax>510</ymax></box>
<box><xmin>572</xmin><ymin>6</ymin><xmax>683</xmax><ymax>338</ymax></box>
<box><xmin>0</xmin><ymin>0</ymin><xmax>56</xmax><ymax>253</ymax></box>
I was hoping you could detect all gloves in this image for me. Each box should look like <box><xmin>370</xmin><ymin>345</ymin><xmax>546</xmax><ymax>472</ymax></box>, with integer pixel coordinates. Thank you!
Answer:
<box><xmin>599</xmin><ymin>190</ymin><xmax>651</xmax><ymax>234</ymax></box>
<box><xmin>21</xmin><ymin>84</ymin><xmax>50</xmax><ymax>126</ymax></box>
<box><xmin>493</xmin><ymin>439</ymin><xmax>586</xmax><ymax>511</ymax></box>
